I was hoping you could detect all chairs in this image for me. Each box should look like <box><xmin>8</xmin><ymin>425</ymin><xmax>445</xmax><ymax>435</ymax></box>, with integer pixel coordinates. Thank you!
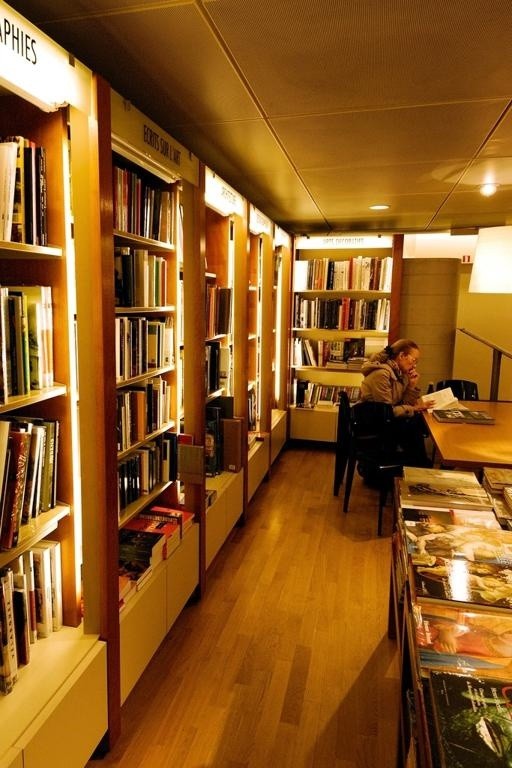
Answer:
<box><xmin>333</xmin><ymin>389</ymin><xmax>359</xmax><ymax>499</ymax></box>
<box><xmin>338</xmin><ymin>400</ymin><xmax>432</xmax><ymax>538</ymax></box>
<box><xmin>436</xmin><ymin>378</ymin><xmax>480</xmax><ymax>400</ymax></box>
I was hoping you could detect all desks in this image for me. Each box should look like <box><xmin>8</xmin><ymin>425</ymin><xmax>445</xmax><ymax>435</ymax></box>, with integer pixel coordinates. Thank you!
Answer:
<box><xmin>419</xmin><ymin>400</ymin><xmax>512</xmax><ymax>471</ymax></box>
<box><xmin>386</xmin><ymin>535</ymin><xmax>512</xmax><ymax>768</ymax></box>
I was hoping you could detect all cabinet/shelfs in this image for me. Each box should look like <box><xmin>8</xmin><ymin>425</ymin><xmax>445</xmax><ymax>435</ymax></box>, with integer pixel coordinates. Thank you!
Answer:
<box><xmin>248</xmin><ymin>201</ymin><xmax>271</xmax><ymax>520</ymax></box>
<box><xmin>270</xmin><ymin>221</ymin><xmax>291</xmax><ymax>483</ymax></box>
<box><xmin>103</xmin><ymin>80</ymin><xmax>203</xmax><ymax>719</ymax></box>
<box><xmin>202</xmin><ymin>159</ymin><xmax>247</xmax><ymax>575</ymax></box>
<box><xmin>291</xmin><ymin>232</ymin><xmax>404</xmax><ymax>444</ymax></box>
<box><xmin>0</xmin><ymin>0</ymin><xmax>117</xmax><ymax>768</ymax></box>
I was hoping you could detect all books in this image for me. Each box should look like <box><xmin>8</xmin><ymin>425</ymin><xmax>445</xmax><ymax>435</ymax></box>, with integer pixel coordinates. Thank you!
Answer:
<box><xmin>422</xmin><ymin>387</ymin><xmax>458</xmax><ymax>414</ymax></box>
<box><xmin>393</xmin><ymin>466</ymin><xmax>512</xmax><ymax>768</ymax></box>
<box><xmin>433</xmin><ymin>410</ymin><xmax>496</xmax><ymax>425</ymax></box>
<box><xmin>290</xmin><ymin>255</ymin><xmax>393</xmax><ymax>409</ymax></box>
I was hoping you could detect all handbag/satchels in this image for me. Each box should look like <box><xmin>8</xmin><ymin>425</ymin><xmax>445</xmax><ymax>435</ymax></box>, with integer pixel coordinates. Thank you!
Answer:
<box><xmin>350</xmin><ymin>401</ymin><xmax>395</xmax><ymax>457</ymax></box>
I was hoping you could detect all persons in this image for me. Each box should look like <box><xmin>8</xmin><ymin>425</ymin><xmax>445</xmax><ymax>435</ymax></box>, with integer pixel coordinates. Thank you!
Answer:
<box><xmin>354</xmin><ymin>338</ymin><xmax>436</xmax><ymax>468</ymax></box>
